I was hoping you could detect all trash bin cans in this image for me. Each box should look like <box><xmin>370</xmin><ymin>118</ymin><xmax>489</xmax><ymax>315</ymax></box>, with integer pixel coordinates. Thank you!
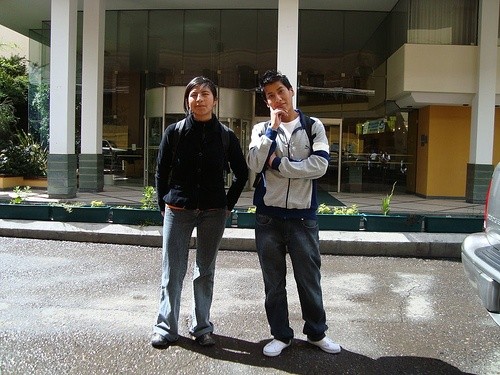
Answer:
<box><xmin>348</xmin><ymin>162</ymin><xmax>364</xmax><ymax>186</ymax></box>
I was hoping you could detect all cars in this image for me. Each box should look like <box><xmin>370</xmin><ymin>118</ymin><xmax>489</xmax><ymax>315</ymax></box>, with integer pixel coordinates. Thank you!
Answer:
<box><xmin>459</xmin><ymin>161</ymin><xmax>500</xmax><ymax>327</ymax></box>
<box><xmin>102</xmin><ymin>138</ymin><xmax>129</xmax><ymax>156</ymax></box>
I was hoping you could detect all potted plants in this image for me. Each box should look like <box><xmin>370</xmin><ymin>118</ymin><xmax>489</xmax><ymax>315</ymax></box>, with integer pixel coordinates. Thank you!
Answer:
<box><xmin>226</xmin><ymin>208</ymin><xmax>236</xmax><ymax>227</ymax></box>
<box><xmin>110</xmin><ymin>185</ymin><xmax>166</xmax><ymax>226</ymax></box>
<box><xmin>316</xmin><ymin>203</ymin><xmax>362</xmax><ymax>230</ymax></box>
<box><xmin>234</xmin><ymin>207</ymin><xmax>258</xmax><ymax>228</ymax></box>
<box><xmin>362</xmin><ymin>181</ymin><xmax>424</xmax><ymax>232</ymax></box>
<box><xmin>0</xmin><ymin>185</ymin><xmax>53</xmax><ymax>221</ymax></box>
<box><xmin>50</xmin><ymin>201</ymin><xmax>110</xmax><ymax>221</ymax></box>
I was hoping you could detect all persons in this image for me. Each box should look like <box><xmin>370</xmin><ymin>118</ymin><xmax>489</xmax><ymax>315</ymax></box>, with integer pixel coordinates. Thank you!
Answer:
<box><xmin>246</xmin><ymin>70</ymin><xmax>341</xmax><ymax>356</ymax></box>
<box><xmin>151</xmin><ymin>77</ymin><xmax>249</xmax><ymax>347</ymax></box>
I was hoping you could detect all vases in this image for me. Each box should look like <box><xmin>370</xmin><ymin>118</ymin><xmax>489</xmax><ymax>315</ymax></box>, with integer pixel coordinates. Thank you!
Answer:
<box><xmin>423</xmin><ymin>213</ymin><xmax>487</xmax><ymax>234</ymax></box>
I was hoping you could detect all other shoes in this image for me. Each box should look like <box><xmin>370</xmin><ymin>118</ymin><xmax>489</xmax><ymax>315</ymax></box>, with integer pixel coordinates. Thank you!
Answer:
<box><xmin>151</xmin><ymin>334</ymin><xmax>179</xmax><ymax>350</ymax></box>
<box><xmin>197</xmin><ymin>333</ymin><xmax>216</xmax><ymax>347</ymax></box>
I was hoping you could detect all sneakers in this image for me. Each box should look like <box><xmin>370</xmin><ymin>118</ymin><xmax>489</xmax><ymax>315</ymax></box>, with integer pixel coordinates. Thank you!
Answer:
<box><xmin>262</xmin><ymin>337</ymin><xmax>292</xmax><ymax>357</ymax></box>
<box><xmin>307</xmin><ymin>335</ymin><xmax>341</xmax><ymax>354</ymax></box>
